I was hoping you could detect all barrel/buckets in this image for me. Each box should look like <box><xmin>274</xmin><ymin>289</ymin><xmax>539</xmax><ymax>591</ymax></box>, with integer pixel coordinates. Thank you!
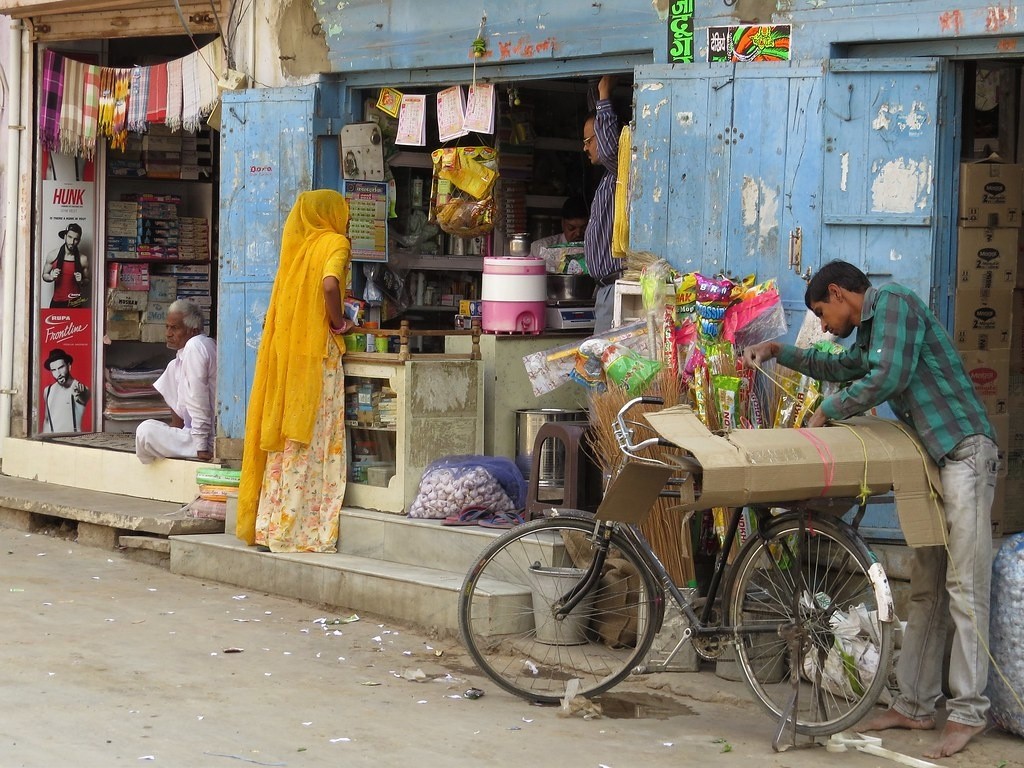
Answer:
<box><xmin>635</xmin><ymin>581</ymin><xmax>700</xmax><ymax>671</ymax></box>
<box><xmin>715</xmin><ymin>598</ymin><xmax>789</xmax><ymax>683</ymax></box>
<box><xmin>528</xmin><ymin>560</ymin><xmax>606</xmax><ymax>645</ymax></box>
<box><xmin>510</xmin><ymin>409</ymin><xmax>589</xmax><ymax>488</ymax></box>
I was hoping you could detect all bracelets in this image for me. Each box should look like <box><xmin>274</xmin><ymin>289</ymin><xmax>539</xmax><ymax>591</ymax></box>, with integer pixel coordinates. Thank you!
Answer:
<box><xmin>330</xmin><ymin>319</ymin><xmax>346</xmax><ymax>334</ymax></box>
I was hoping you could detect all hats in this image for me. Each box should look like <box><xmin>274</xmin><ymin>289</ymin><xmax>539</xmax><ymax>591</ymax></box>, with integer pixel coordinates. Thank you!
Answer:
<box><xmin>58</xmin><ymin>223</ymin><xmax>81</xmax><ymax>239</ymax></box>
<box><xmin>45</xmin><ymin>349</ymin><xmax>74</xmax><ymax>370</ymax></box>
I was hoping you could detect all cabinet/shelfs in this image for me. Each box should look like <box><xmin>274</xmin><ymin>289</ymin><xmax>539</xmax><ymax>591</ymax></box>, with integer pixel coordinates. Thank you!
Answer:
<box><xmin>338</xmin><ymin>360</ymin><xmax>486</xmax><ymax>518</ymax></box>
<box><xmin>105</xmin><ymin>132</ymin><xmax>220</xmax><ymax>431</ymax></box>
<box><xmin>365</xmin><ymin>149</ymin><xmax>503</xmax><ymax>309</ymax></box>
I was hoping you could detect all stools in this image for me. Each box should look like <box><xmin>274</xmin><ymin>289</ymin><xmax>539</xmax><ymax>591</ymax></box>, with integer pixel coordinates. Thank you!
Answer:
<box><xmin>524</xmin><ymin>421</ymin><xmax>602</xmax><ymax>524</ymax></box>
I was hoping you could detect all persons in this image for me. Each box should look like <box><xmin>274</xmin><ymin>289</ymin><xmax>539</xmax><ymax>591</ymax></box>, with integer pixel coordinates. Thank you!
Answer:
<box><xmin>42</xmin><ymin>349</ymin><xmax>89</xmax><ymax>433</ymax></box>
<box><xmin>583</xmin><ymin>74</ymin><xmax>629</xmax><ymax>335</ymax></box>
<box><xmin>742</xmin><ymin>260</ymin><xmax>1000</xmax><ymax>758</ymax></box>
<box><xmin>135</xmin><ymin>298</ymin><xmax>217</xmax><ymax>464</ymax></box>
<box><xmin>237</xmin><ymin>190</ymin><xmax>354</xmax><ymax>555</ymax></box>
<box><xmin>530</xmin><ymin>196</ymin><xmax>591</xmax><ymax>256</ymax></box>
<box><xmin>42</xmin><ymin>223</ymin><xmax>89</xmax><ymax>309</ymax></box>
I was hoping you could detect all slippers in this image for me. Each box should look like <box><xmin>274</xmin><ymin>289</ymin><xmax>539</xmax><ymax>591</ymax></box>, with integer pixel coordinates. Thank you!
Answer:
<box><xmin>477</xmin><ymin>512</ymin><xmax>525</xmax><ymax>527</ymax></box>
<box><xmin>441</xmin><ymin>508</ymin><xmax>496</xmax><ymax>526</ymax></box>
<box><xmin>446</xmin><ymin>506</ymin><xmax>496</xmax><ymax>519</ymax></box>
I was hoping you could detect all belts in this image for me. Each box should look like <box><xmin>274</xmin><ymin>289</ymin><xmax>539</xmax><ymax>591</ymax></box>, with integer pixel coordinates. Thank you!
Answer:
<box><xmin>594</xmin><ymin>271</ymin><xmax>622</xmax><ymax>287</ymax></box>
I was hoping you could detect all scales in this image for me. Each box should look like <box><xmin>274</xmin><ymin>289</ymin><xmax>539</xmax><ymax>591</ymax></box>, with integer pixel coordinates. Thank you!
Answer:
<box><xmin>545</xmin><ymin>298</ymin><xmax>596</xmax><ymax>334</ymax></box>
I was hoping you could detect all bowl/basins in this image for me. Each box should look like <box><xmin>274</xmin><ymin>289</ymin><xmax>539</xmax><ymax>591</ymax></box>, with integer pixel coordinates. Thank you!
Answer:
<box><xmin>547</xmin><ymin>274</ymin><xmax>598</xmax><ymax>300</ymax></box>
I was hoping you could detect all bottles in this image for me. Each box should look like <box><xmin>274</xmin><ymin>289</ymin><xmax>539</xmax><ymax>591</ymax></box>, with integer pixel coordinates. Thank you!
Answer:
<box><xmin>375</xmin><ymin>335</ymin><xmax>388</xmax><ymax>353</ymax></box>
<box><xmin>509</xmin><ymin>233</ymin><xmax>531</xmax><ymax>257</ymax></box>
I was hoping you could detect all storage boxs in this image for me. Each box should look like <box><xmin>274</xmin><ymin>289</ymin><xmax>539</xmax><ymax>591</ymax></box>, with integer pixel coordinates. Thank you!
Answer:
<box><xmin>990</xmin><ymin>476</ymin><xmax>1024</xmax><ymax>538</ymax></box>
<box><xmin>958</xmin><ymin>227</ymin><xmax>1024</xmax><ymax>289</ymax></box>
<box><xmin>957</xmin><ymin>351</ymin><xmax>1024</xmax><ymax>415</ymax></box>
<box><xmin>105</xmin><ymin>123</ymin><xmax>211</xmax><ymax>342</ymax></box>
<box><xmin>989</xmin><ymin>415</ymin><xmax>1024</xmax><ymax>475</ymax></box>
<box><xmin>955</xmin><ymin>289</ymin><xmax>1024</xmax><ymax>349</ymax></box>
<box><xmin>957</xmin><ymin>153</ymin><xmax>1024</xmax><ymax>227</ymax></box>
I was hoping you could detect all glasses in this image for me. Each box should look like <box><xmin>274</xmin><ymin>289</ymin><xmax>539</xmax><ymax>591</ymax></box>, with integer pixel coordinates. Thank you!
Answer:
<box><xmin>583</xmin><ymin>134</ymin><xmax>596</xmax><ymax>145</ymax></box>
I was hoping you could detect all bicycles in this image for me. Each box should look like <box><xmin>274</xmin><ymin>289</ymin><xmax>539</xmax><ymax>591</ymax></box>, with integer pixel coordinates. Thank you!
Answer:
<box><xmin>456</xmin><ymin>396</ymin><xmax>896</xmax><ymax>753</ymax></box>
